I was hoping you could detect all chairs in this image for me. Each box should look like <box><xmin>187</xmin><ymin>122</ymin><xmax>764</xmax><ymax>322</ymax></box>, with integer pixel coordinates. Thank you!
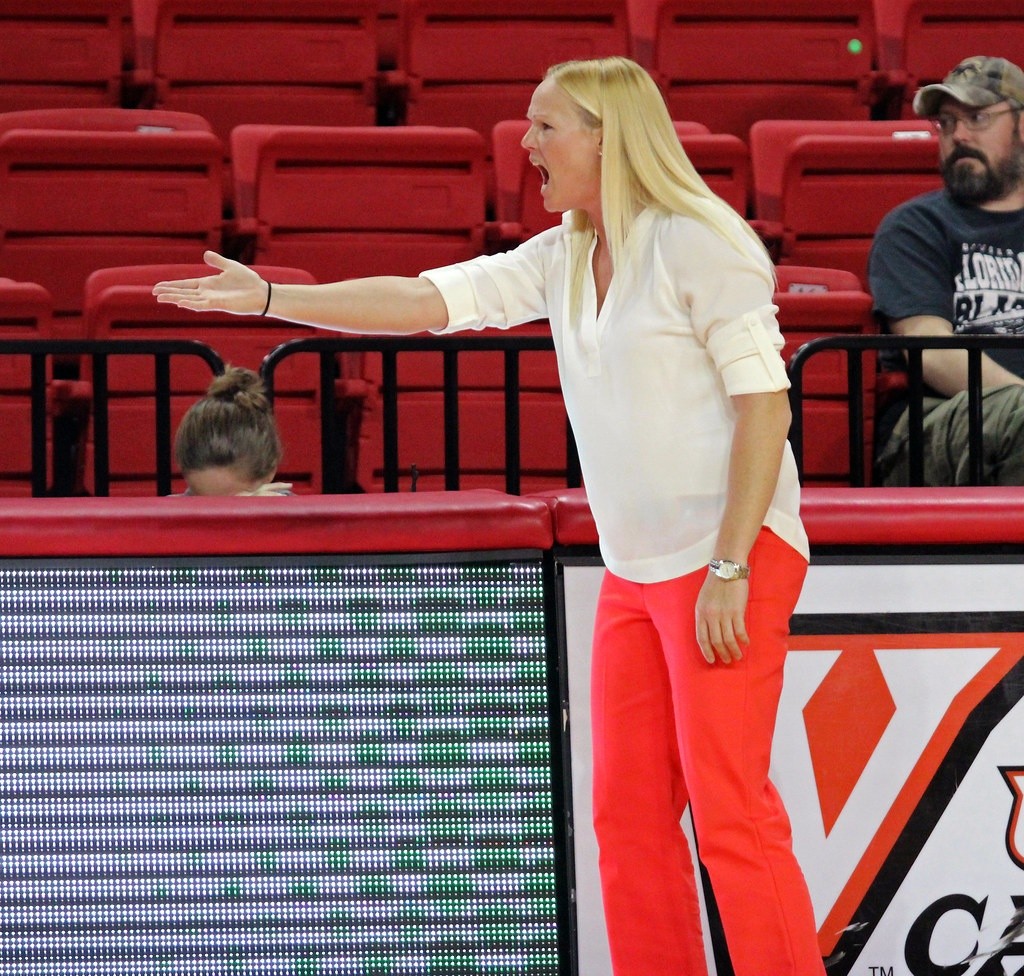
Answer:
<box><xmin>0</xmin><ymin>1</ymin><xmax>1024</xmax><ymax>489</ymax></box>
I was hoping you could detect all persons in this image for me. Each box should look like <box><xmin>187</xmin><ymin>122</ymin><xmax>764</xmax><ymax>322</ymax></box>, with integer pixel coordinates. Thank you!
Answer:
<box><xmin>164</xmin><ymin>362</ymin><xmax>299</xmax><ymax>499</ymax></box>
<box><xmin>151</xmin><ymin>58</ymin><xmax>831</xmax><ymax>976</ymax></box>
<box><xmin>869</xmin><ymin>55</ymin><xmax>1024</xmax><ymax>486</ymax></box>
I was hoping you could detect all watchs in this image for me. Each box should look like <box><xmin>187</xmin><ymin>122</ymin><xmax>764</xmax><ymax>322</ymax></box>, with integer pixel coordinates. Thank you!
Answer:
<box><xmin>709</xmin><ymin>558</ymin><xmax>750</xmax><ymax>581</ymax></box>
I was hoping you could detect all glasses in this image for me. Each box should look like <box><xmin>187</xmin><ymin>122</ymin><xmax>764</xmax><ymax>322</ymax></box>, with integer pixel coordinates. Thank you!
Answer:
<box><xmin>927</xmin><ymin>106</ymin><xmax>1023</xmax><ymax>130</ymax></box>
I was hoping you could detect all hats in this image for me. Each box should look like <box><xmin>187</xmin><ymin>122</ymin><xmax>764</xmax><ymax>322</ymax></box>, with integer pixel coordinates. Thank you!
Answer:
<box><xmin>913</xmin><ymin>56</ymin><xmax>1024</xmax><ymax>114</ymax></box>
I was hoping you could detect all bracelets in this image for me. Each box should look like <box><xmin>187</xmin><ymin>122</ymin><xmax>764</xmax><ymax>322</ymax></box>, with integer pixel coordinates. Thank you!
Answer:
<box><xmin>261</xmin><ymin>281</ymin><xmax>271</xmax><ymax>318</ymax></box>
